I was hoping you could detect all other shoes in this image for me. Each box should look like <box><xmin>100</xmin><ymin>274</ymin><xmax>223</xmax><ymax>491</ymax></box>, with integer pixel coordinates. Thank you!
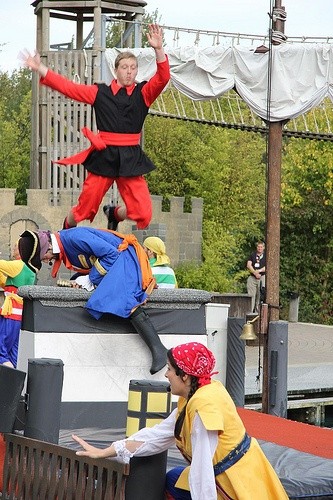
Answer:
<box><xmin>103</xmin><ymin>205</ymin><xmax>121</xmax><ymax>231</ymax></box>
<box><xmin>62</xmin><ymin>216</ymin><xmax>76</xmax><ymax>231</ymax></box>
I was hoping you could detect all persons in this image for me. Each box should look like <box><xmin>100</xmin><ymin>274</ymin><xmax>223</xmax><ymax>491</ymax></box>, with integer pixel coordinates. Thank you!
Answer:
<box><xmin>246</xmin><ymin>242</ymin><xmax>266</xmax><ymax>313</ymax></box>
<box><xmin>143</xmin><ymin>237</ymin><xmax>177</xmax><ymax>289</ymax></box>
<box><xmin>0</xmin><ymin>237</ymin><xmax>36</xmax><ymax>369</ymax></box>
<box><xmin>72</xmin><ymin>342</ymin><xmax>289</xmax><ymax>500</ymax></box>
<box><xmin>18</xmin><ymin>22</ymin><xmax>171</xmax><ymax>229</ymax></box>
<box><xmin>18</xmin><ymin>226</ymin><xmax>170</xmax><ymax>375</ymax></box>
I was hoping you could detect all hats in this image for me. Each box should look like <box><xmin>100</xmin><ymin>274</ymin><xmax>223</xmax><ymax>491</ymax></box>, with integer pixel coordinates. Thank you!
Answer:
<box><xmin>17</xmin><ymin>230</ymin><xmax>41</xmax><ymax>274</ymax></box>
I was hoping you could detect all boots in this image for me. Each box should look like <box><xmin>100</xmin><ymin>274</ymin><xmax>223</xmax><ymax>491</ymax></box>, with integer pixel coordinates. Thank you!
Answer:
<box><xmin>129</xmin><ymin>306</ymin><xmax>169</xmax><ymax>375</ymax></box>
